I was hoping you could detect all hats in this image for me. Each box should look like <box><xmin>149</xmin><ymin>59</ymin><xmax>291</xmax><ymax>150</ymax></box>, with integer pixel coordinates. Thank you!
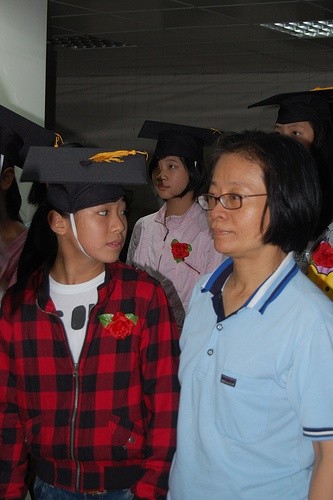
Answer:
<box><xmin>0</xmin><ymin>104</ymin><xmax>64</xmax><ymax>170</ymax></box>
<box><xmin>136</xmin><ymin>119</ymin><xmax>224</xmax><ymax>157</ymax></box>
<box><xmin>18</xmin><ymin>146</ymin><xmax>152</xmax><ymax>214</ymax></box>
<box><xmin>247</xmin><ymin>86</ymin><xmax>333</xmax><ymax>125</ymax></box>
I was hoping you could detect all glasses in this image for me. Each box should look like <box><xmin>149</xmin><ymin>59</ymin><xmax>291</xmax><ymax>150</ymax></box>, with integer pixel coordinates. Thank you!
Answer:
<box><xmin>196</xmin><ymin>192</ymin><xmax>269</xmax><ymax>210</ymax></box>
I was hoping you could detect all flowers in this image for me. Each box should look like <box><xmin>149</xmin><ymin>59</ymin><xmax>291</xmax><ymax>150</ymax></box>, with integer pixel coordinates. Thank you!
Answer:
<box><xmin>309</xmin><ymin>240</ymin><xmax>333</xmax><ymax>268</ymax></box>
<box><xmin>170</xmin><ymin>239</ymin><xmax>193</xmax><ymax>264</ymax></box>
<box><xmin>99</xmin><ymin>312</ymin><xmax>139</xmax><ymax>340</ymax></box>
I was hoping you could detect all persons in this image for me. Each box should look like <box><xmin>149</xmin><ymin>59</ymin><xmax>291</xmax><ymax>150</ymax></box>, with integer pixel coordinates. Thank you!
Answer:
<box><xmin>125</xmin><ymin>119</ymin><xmax>232</xmax><ymax>315</ymax></box>
<box><xmin>246</xmin><ymin>87</ymin><xmax>333</xmax><ymax>301</ymax></box>
<box><xmin>0</xmin><ymin>145</ymin><xmax>181</xmax><ymax>500</ymax></box>
<box><xmin>169</xmin><ymin>130</ymin><xmax>333</xmax><ymax>500</ymax></box>
